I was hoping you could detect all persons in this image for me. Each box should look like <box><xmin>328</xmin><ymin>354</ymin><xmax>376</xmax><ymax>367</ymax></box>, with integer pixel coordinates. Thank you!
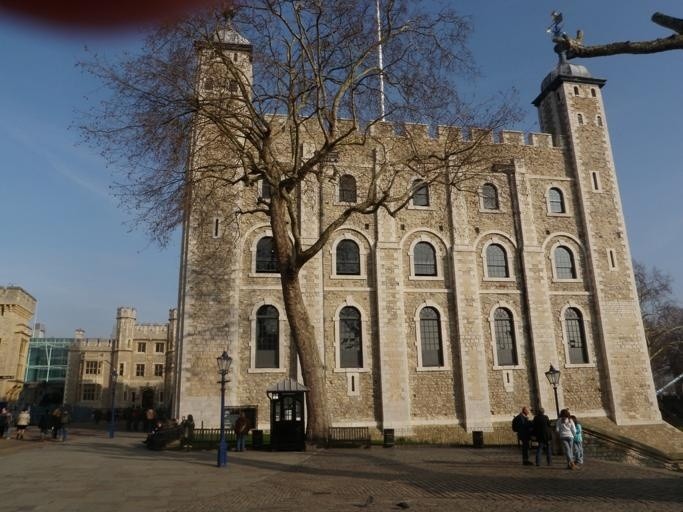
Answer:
<box><xmin>0</xmin><ymin>404</ymin><xmax>71</xmax><ymax>443</ymax></box>
<box><xmin>124</xmin><ymin>405</ymin><xmax>156</xmax><ymax>431</ymax></box>
<box><xmin>555</xmin><ymin>408</ymin><xmax>581</xmax><ymax>470</ymax></box>
<box><xmin>511</xmin><ymin>406</ymin><xmax>533</xmax><ymax>465</ymax></box>
<box><xmin>570</xmin><ymin>414</ymin><xmax>583</xmax><ymax>465</ymax></box>
<box><xmin>141</xmin><ymin>413</ymin><xmax>197</xmax><ymax>450</ymax></box>
<box><xmin>233</xmin><ymin>411</ymin><xmax>249</xmax><ymax>452</ymax></box>
<box><xmin>530</xmin><ymin>406</ymin><xmax>554</xmax><ymax>466</ymax></box>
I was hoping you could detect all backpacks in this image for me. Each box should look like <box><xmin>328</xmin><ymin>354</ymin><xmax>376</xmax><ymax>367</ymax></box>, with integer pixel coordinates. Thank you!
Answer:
<box><xmin>510</xmin><ymin>415</ymin><xmax>525</xmax><ymax>431</ymax></box>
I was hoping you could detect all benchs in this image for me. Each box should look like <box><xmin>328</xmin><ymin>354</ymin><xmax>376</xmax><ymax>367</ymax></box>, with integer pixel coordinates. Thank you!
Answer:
<box><xmin>323</xmin><ymin>426</ymin><xmax>372</xmax><ymax>450</ymax></box>
<box><xmin>180</xmin><ymin>428</ymin><xmax>247</xmax><ymax>451</ymax></box>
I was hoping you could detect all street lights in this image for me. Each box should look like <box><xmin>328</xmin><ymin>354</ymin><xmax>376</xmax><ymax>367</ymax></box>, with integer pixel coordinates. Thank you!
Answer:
<box><xmin>214</xmin><ymin>350</ymin><xmax>231</xmax><ymax>468</ymax></box>
<box><xmin>108</xmin><ymin>367</ymin><xmax>119</xmax><ymax>438</ymax></box>
<box><xmin>544</xmin><ymin>362</ymin><xmax>560</xmax><ymax>419</ymax></box>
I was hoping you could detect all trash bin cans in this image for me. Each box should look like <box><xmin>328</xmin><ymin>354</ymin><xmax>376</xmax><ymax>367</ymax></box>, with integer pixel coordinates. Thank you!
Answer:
<box><xmin>473</xmin><ymin>431</ymin><xmax>484</xmax><ymax>449</ymax></box>
<box><xmin>253</xmin><ymin>430</ymin><xmax>263</xmax><ymax>451</ymax></box>
<box><xmin>383</xmin><ymin>429</ymin><xmax>394</xmax><ymax>448</ymax></box>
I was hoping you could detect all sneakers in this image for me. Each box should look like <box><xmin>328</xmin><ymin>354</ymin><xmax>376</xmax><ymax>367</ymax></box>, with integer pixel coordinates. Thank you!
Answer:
<box><xmin>520</xmin><ymin>459</ymin><xmax>584</xmax><ymax>471</ymax></box>
<box><xmin>2</xmin><ymin>435</ymin><xmax>67</xmax><ymax>444</ymax></box>
